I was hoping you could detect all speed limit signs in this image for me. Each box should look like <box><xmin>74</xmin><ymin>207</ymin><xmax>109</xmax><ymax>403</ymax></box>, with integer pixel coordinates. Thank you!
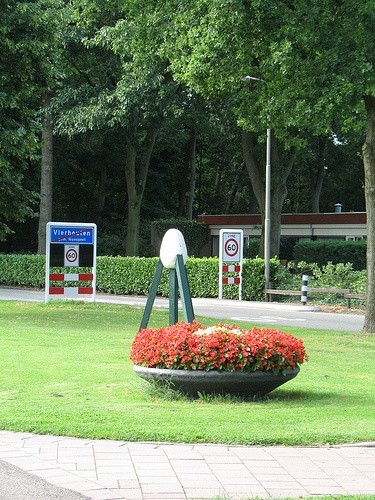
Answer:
<box><xmin>64</xmin><ymin>244</ymin><xmax>79</xmax><ymax>267</ymax></box>
<box><xmin>223</xmin><ymin>233</ymin><xmax>240</xmax><ymax>262</ymax></box>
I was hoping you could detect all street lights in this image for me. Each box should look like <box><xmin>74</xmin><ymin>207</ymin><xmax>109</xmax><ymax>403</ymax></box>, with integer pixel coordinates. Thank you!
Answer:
<box><xmin>244</xmin><ymin>75</ymin><xmax>275</xmax><ymax>301</ymax></box>
